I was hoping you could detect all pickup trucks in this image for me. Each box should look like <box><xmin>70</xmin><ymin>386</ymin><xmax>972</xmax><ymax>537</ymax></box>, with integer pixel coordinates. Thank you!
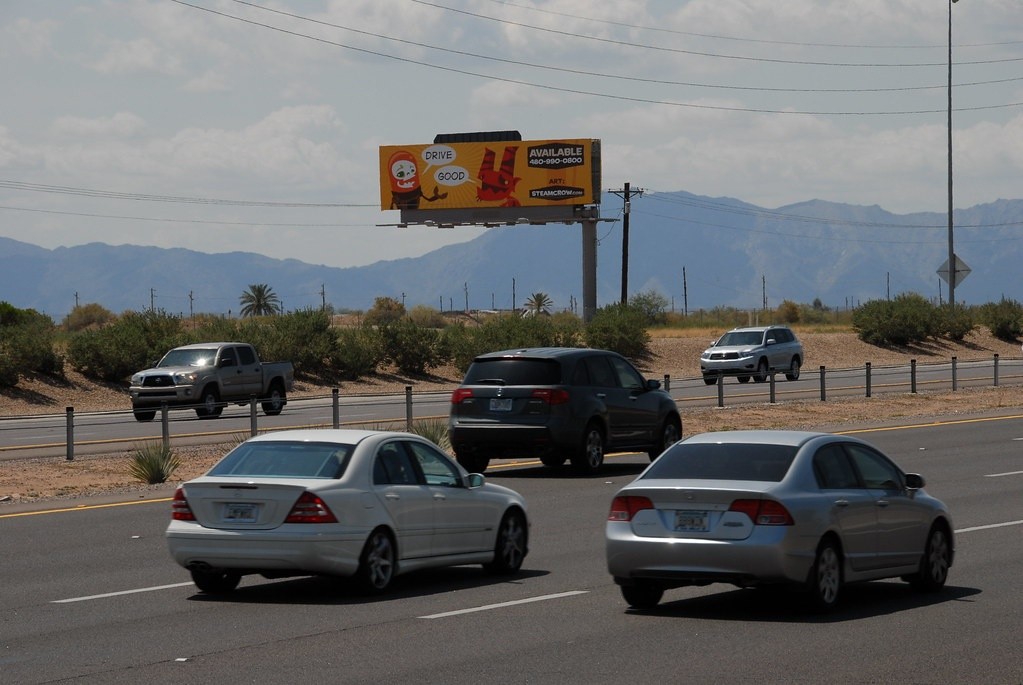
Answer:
<box><xmin>128</xmin><ymin>341</ymin><xmax>294</xmax><ymax>423</ymax></box>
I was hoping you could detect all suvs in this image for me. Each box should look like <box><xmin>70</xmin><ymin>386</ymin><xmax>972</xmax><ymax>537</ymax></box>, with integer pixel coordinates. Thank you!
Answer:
<box><xmin>700</xmin><ymin>325</ymin><xmax>804</xmax><ymax>385</ymax></box>
<box><xmin>448</xmin><ymin>347</ymin><xmax>682</xmax><ymax>477</ymax></box>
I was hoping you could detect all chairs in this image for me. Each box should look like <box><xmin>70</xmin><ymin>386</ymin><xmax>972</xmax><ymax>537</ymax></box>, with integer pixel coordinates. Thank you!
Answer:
<box><xmin>382</xmin><ymin>448</ymin><xmax>404</xmax><ymax>485</ymax></box>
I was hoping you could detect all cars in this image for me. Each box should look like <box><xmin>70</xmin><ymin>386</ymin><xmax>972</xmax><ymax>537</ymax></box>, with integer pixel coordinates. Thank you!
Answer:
<box><xmin>166</xmin><ymin>427</ymin><xmax>532</xmax><ymax>601</ymax></box>
<box><xmin>604</xmin><ymin>429</ymin><xmax>957</xmax><ymax>616</ymax></box>
